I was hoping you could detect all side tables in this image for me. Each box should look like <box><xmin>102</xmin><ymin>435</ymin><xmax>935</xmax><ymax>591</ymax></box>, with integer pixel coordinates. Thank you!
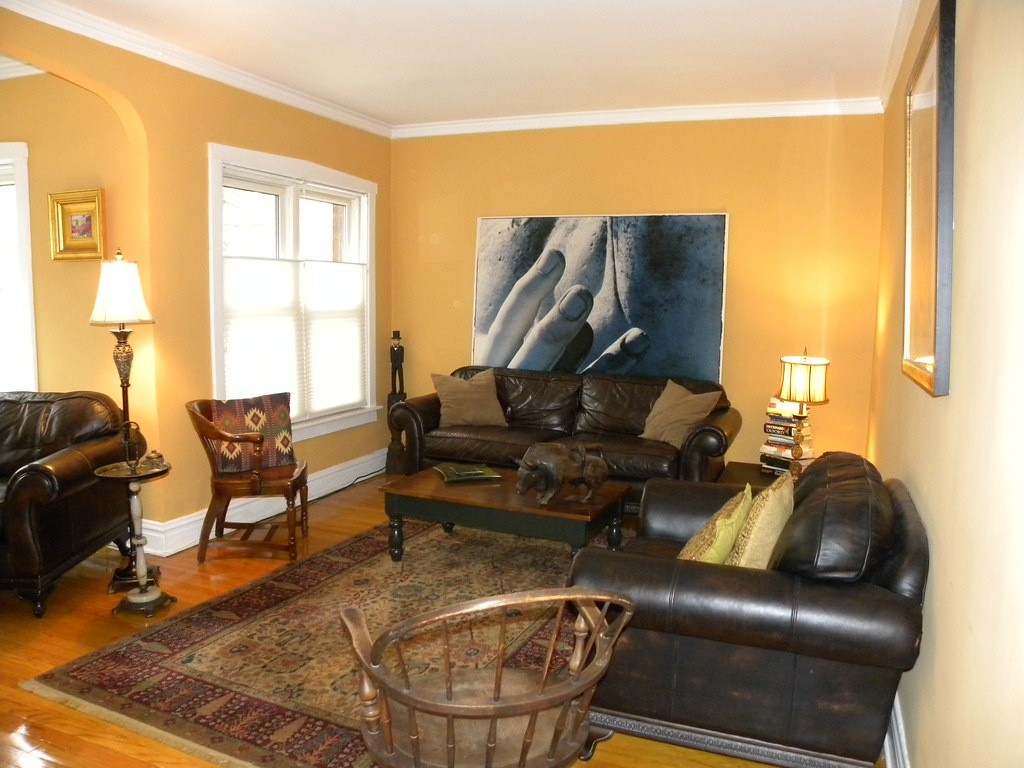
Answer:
<box><xmin>716</xmin><ymin>460</ymin><xmax>780</xmax><ymax>487</ymax></box>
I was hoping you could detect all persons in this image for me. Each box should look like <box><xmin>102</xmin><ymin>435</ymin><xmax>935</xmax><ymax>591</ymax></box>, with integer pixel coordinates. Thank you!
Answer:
<box><xmin>478</xmin><ymin>249</ymin><xmax>652</xmax><ymax>372</ymax></box>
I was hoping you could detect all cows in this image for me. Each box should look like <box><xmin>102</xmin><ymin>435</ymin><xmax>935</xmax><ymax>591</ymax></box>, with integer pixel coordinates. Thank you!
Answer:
<box><xmin>510</xmin><ymin>450</ymin><xmax>608</xmax><ymax>505</ymax></box>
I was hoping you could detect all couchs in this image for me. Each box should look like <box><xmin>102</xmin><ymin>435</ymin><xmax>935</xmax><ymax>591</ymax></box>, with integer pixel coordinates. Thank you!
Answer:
<box><xmin>0</xmin><ymin>391</ymin><xmax>148</xmax><ymax>619</ymax></box>
<box><xmin>565</xmin><ymin>451</ymin><xmax>930</xmax><ymax>768</ymax></box>
<box><xmin>389</xmin><ymin>365</ymin><xmax>742</xmax><ymax>513</ymax></box>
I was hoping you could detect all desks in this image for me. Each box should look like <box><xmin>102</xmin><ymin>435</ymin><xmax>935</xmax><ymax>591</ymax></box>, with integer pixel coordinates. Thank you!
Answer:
<box><xmin>94</xmin><ymin>460</ymin><xmax>177</xmax><ymax>619</ymax></box>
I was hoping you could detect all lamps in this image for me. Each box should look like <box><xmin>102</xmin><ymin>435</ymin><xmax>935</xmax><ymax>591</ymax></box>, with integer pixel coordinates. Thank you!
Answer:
<box><xmin>89</xmin><ymin>247</ymin><xmax>155</xmax><ymax>459</ymax></box>
<box><xmin>774</xmin><ymin>347</ymin><xmax>829</xmax><ymax>482</ymax></box>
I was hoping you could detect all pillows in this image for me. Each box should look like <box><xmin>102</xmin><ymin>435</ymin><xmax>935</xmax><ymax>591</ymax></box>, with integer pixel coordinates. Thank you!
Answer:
<box><xmin>723</xmin><ymin>469</ymin><xmax>795</xmax><ymax>570</ymax></box>
<box><xmin>430</xmin><ymin>367</ymin><xmax>509</xmax><ymax>428</ymax></box>
<box><xmin>676</xmin><ymin>481</ymin><xmax>752</xmax><ymax>564</ymax></box>
<box><xmin>637</xmin><ymin>379</ymin><xmax>722</xmax><ymax>450</ymax></box>
<box><xmin>211</xmin><ymin>392</ymin><xmax>295</xmax><ymax>473</ymax></box>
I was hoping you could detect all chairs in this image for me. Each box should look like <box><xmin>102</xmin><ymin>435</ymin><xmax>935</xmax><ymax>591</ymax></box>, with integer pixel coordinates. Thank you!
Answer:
<box><xmin>340</xmin><ymin>586</ymin><xmax>634</xmax><ymax>768</ymax></box>
<box><xmin>185</xmin><ymin>399</ymin><xmax>309</xmax><ymax>564</ymax></box>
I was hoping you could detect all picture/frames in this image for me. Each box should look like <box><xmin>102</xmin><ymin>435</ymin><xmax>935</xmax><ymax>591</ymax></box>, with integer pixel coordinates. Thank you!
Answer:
<box><xmin>47</xmin><ymin>188</ymin><xmax>104</xmax><ymax>262</ymax></box>
<box><xmin>903</xmin><ymin>0</ymin><xmax>956</xmax><ymax>398</ymax></box>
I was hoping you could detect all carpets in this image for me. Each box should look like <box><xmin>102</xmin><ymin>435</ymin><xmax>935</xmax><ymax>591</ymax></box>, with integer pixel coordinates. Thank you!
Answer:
<box><xmin>17</xmin><ymin>518</ymin><xmax>636</xmax><ymax>768</ymax></box>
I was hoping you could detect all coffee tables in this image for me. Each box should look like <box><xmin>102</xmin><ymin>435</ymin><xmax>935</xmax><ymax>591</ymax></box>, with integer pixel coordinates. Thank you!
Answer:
<box><xmin>378</xmin><ymin>463</ymin><xmax>631</xmax><ymax>561</ymax></box>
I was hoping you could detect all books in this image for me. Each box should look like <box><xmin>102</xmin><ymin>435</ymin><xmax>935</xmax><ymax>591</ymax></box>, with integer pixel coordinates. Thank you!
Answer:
<box><xmin>759</xmin><ymin>397</ymin><xmax>817</xmax><ymax>481</ymax></box>
